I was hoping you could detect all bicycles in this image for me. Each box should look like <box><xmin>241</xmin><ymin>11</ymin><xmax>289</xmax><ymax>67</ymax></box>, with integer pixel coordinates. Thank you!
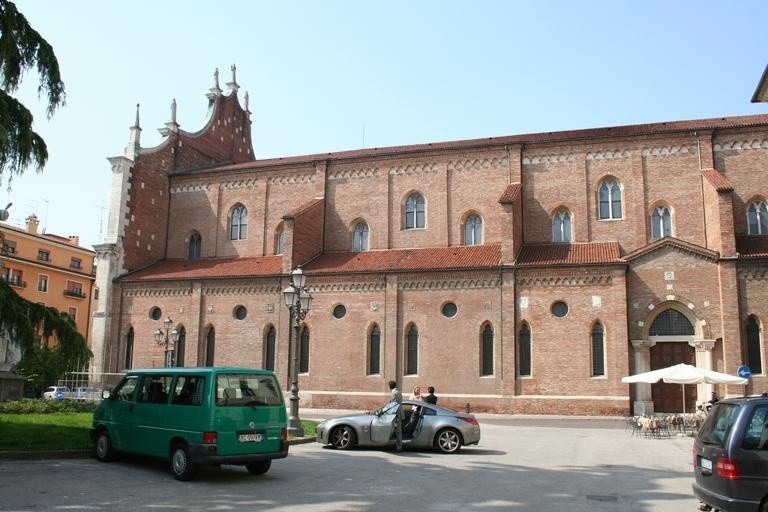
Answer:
<box><xmin>694</xmin><ymin>401</ymin><xmax>712</xmax><ymax>423</ymax></box>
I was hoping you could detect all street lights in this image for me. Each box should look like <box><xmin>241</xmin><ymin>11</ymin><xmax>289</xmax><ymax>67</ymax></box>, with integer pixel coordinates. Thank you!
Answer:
<box><xmin>282</xmin><ymin>264</ymin><xmax>314</xmax><ymax>437</ymax></box>
<box><xmin>154</xmin><ymin>317</ymin><xmax>180</xmax><ymax>368</ymax></box>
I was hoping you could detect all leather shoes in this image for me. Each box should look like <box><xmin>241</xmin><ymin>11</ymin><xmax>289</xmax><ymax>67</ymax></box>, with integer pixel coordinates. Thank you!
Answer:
<box><xmin>392</xmin><ymin>449</ymin><xmax>400</xmax><ymax>452</ymax></box>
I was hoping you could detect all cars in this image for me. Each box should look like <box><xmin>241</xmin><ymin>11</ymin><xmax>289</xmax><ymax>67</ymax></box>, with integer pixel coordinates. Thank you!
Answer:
<box><xmin>314</xmin><ymin>400</ymin><xmax>480</xmax><ymax>454</ymax></box>
<box><xmin>43</xmin><ymin>386</ymin><xmax>87</xmax><ymax>402</ymax></box>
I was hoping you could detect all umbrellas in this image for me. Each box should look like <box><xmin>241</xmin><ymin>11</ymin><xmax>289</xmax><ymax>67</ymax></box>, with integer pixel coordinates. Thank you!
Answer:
<box><xmin>620</xmin><ymin>360</ymin><xmax>752</xmax><ymax>415</ymax></box>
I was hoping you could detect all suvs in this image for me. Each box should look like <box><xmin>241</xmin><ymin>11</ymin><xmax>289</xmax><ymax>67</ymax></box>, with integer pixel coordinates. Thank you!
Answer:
<box><xmin>692</xmin><ymin>392</ymin><xmax>768</xmax><ymax>512</ymax></box>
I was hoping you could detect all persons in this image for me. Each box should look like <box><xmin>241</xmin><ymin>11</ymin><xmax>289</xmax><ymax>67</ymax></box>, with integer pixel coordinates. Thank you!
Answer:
<box><xmin>423</xmin><ymin>387</ymin><xmax>438</xmax><ymax>415</ymax></box>
<box><xmin>701</xmin><ymin>392</ymin><xmax>719</xmax><ymax>414</ymax></box>
<box><xmin>387</xmin><ymin>379</ymin><xmax>403</xmax><ymax>405</ymax></box>
<box><xmin>409</xmin><ymin>385</ymin><xmax>424</xmax><ymax>413</ymax></box>
<box><xmin>389</xmin><ymin>404</ymin><xmax>406</xmax><ymax>453</ymax></box>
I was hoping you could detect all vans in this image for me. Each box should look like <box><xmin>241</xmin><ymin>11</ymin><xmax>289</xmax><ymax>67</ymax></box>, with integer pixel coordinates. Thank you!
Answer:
<box><xmin>93</xmin><ymin>367</ymin><xmax>289</xmax><ymax>481</ymax></box>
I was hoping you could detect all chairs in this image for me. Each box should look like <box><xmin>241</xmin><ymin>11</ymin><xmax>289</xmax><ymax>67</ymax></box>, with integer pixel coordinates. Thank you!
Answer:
<box><xmin>712</xmin><ymin>417</ymin><xmax>726</xmax><ymax>442</ymax></box>
<box><xmin>146</xmin><ymin>382</ymin><xmax>236</xmax><ymax>406</ymax></box>
<box><xmin>402</xmin><ymin>412</ymin><xmax>418</xmax><ymax>430</ymax></box>
<box><xmin>623</xmin><ymin>408</ymin><xmax>702</xmax><ymax>439</ymax></box>
